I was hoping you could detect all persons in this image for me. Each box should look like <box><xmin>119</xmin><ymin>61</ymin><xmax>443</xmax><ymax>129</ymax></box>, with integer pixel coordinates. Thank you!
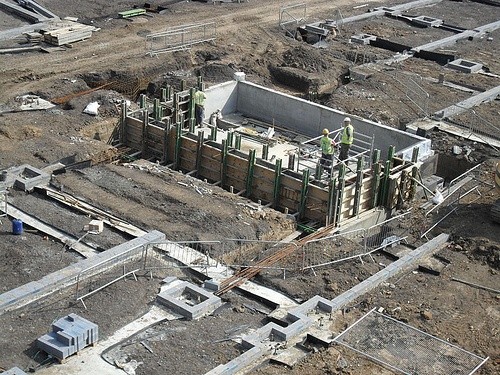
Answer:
<box><xmin>192</xmin><ymin>85</ymin><xmax>206</xmax><ymax>128</ymax></box>
<box><xmin>319</xmin><ymin>128</ymin><xmax>342</xmax><ymax>179</ymax></box>
<box><xmin>335</xmin><ymin>117</ymin><xmax>353</xmax><ymax>169</ymax></box>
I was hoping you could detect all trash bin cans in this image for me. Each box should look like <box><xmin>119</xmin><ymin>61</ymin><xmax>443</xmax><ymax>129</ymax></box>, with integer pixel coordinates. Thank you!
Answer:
<box><xmin>12</xmin><ymin>219</ymin><xmax>23</xmax><ymax>235</ymax></box>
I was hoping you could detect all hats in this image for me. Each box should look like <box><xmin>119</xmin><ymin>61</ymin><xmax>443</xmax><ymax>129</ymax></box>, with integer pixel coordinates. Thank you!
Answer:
<box><xmin>196</xmin><ymin>87</ymin><xmax>199</xmax><ymax>89</ymax></box>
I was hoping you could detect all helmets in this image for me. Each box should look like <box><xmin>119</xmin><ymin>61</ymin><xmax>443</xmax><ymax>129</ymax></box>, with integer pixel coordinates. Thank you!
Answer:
<box><xmin>321</xmin><ymin>128</ymin><xmax>329</xmax><ymax>134</ymax></box>
<box><xmin>343</xmin><ymin>117</ymin><xmax>350</xmax><ymax>122</ymax></box>
